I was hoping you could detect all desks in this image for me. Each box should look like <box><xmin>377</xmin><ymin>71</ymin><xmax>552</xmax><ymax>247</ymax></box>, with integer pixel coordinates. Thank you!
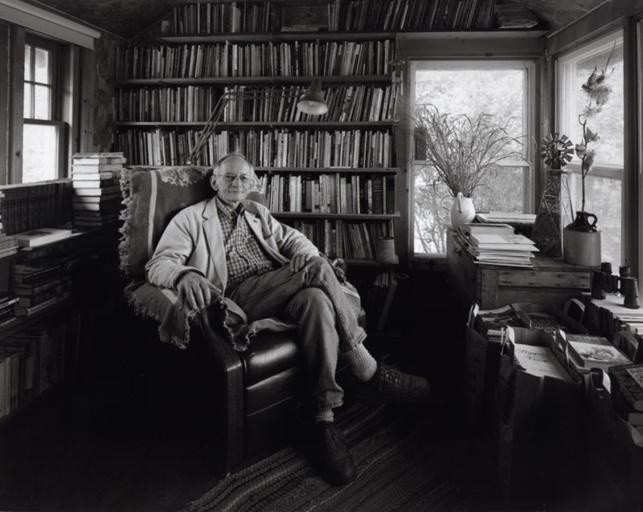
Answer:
<box><xmin>444</xmin><ymin>229</ymin><xmax>593</xmax><ymax>310</ymax></box>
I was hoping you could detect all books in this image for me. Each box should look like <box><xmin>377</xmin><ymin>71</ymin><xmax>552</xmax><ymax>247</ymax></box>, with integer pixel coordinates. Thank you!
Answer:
<box><xmin>101</xmin><ymin>1</ymin><xmax>400</xmax><ymax>122</ymax></box>
<box><xmin>117</xmin><ymin>127</ymin><xmax>401</xmax><ymax>261</ymax></box>
<box><xmin>461</xmin><ymin>285</ymin><xmax>642</xmax><ymax>442</ymax></box>
<box><xmin>454</xmin><ymin>210</ymin><xmax>540</xmax><ymax>270</ymax></box>
<box><xmin>0</xmin><ymin>152</ymin><xmax>107</xmax><ymax>421</ymax></box>
<box><xmin>400</xmin><ymin>0</ymin><xmax>540</xmax><ymax>31</ymax></box>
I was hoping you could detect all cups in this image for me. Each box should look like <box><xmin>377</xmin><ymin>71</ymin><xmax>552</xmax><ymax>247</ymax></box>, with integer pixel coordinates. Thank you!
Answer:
<box><xmin>375</xmin><ymin>236</ymin><xmax>395</xmax><ymax>264</ymax></box>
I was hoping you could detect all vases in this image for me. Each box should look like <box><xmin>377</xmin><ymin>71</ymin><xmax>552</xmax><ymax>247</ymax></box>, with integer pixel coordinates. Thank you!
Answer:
<box><xmin>561</xmin><ymin>211</ymin><xmax>601</xmax><ymax>268</ymax></box>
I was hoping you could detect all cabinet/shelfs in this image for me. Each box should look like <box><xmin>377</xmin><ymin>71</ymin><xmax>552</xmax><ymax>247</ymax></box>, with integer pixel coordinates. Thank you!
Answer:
<box><xmin>113</xmin><ymin>30</ymin><xmax>400</xmax><ymax>304</ymax></box>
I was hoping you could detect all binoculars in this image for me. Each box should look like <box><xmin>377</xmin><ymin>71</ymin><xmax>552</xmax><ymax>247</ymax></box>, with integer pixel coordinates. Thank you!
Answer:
<box><xmin>590</xmin><ymin>263</ymin><xmax>640</xmax><ymax>309</ymax></box>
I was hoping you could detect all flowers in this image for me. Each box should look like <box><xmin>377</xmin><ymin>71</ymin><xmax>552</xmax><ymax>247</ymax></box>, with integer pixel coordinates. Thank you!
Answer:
<box><xmin>568</xmin><ymin>39</ymin><xmax>618</xmax><ymax>213</ymax></box>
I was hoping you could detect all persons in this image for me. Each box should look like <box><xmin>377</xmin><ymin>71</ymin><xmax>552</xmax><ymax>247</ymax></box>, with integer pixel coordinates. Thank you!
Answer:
<box><xmin>143</xmin><ymin>151</ymin><xmax>434</xmax><ymax>486</ymax></box>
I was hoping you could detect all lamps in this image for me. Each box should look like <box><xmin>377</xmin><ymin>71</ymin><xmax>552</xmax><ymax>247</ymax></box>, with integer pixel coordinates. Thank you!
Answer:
<box><xmin>187</xmin><ymin>77</ymin><xmax>330</xmax><ymax>162</ymax></box>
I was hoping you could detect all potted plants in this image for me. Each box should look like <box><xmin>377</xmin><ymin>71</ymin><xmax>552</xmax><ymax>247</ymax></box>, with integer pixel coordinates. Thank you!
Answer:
<box><xmin>403</xmin><ymin>99</ymin><xmax>521</xmax><ymax>226</ymax></box>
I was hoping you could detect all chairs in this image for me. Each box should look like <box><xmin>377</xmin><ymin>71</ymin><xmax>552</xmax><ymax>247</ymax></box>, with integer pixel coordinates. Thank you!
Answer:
<box><xmin>120</xmin><ymin>165</ymin><xmax>306</xmax><ymax>475</ymax></box>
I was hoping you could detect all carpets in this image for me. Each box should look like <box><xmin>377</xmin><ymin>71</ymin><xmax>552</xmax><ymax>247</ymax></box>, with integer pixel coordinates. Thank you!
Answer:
<box><xmin>185</xmin><ymin>397</ymin><xmax>495</xmax><ymax>512</ymax></box>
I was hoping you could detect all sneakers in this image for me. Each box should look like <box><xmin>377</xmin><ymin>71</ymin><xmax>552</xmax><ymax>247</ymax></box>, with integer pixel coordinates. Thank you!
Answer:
<box><xmin>353</xmin><ymin>361</ymin><xmax>431</xmax><ymax>403</ymax></box>
<box><xmin>315</xmin><ymin>419</ymin><xmax>357</xmax><ymax>485</ymax></box>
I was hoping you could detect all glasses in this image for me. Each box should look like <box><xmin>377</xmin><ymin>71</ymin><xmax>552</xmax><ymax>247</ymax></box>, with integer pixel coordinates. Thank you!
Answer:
<box><xmin>214</xmin><ymin>173</ymin><xmax>250</xmax><ymax>182</ymax></box>
